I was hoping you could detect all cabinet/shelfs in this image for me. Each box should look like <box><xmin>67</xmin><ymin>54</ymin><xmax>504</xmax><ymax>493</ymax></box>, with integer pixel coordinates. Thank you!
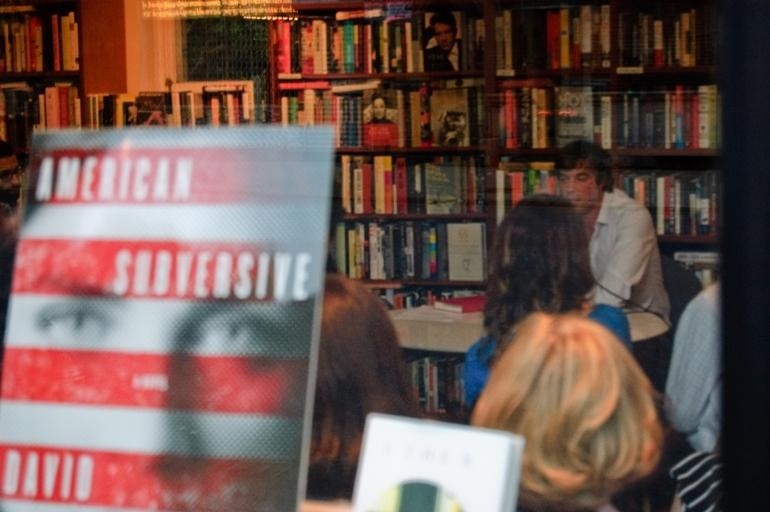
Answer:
<box><xmin>270</xmin><ymin>1</ymin><xmax>490</xmax><ymax>425</ymax></box>
<box><xmin>1</xmin><ymin>1</ymin><xmax>89</xmax><ymax>167</ymax></box>
<box><xmin>489</xmin><ymin>1</ymin><xmax>721</xmax><ymax>246</ymax></box>
